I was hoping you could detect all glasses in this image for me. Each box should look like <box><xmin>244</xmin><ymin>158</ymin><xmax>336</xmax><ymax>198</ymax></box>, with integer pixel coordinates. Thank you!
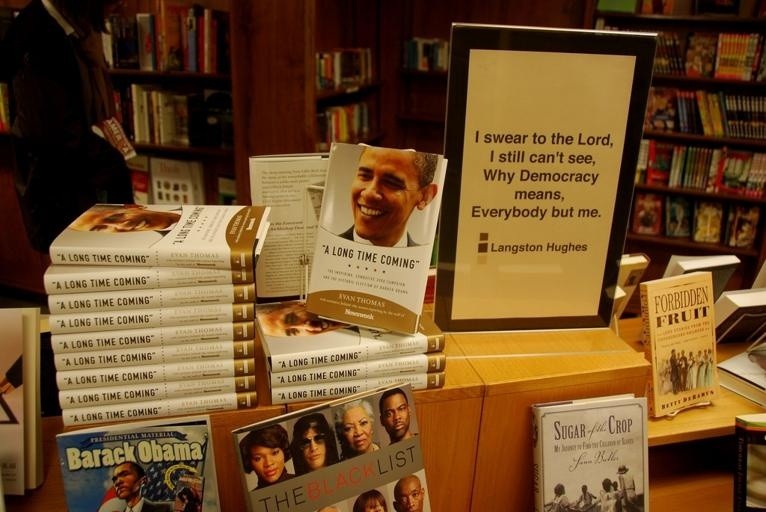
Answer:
<box><xmin>299</xmin><ymin>434</ymin><xmax>326</xmax><ymax>449</ymax></box>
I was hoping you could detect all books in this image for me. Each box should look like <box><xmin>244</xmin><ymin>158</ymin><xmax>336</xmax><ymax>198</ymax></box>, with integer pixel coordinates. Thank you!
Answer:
<box><xmin>305</xmin><ymin>142</ymin><xmax>448</xmax><ymax>336</ymax></box>
<box><xmin>42</xmin><ymin>264</ymin><xmax>275</xmax><ymax>427</ymax></box>
<box><xmin>257</xmin><ymin>300</ymin><xmax>447</xmax><ymax>406</ymax></box>
<box><xmin>401</xmin><ymin>36</ymin><xmax>448</xmax><ymax>73</ymax></box>
<box><xmin>591</xmin><ymin>1</ymin><xmax>766</xmax><ymax>419</ymax></box>
<box><xmin>56</xmin><ymin>414</ymin><xmax>221</xmax><ymax>511</ymax></box>
<box><xmin>231</xmin><ymin>382</ymin><xmax>433</xmax><ymax>512</ymax></box>
<box><xmin>2</xmin><ymin>2</ymin><xmax>233</xmax><ymax>206</ymax></box>
<box><xmin>313</xmin><ymin>46</ymin><xmax>372</xmax><ymax>153</ymax></box>
<box><xmin>0</xmin><ymin>308</ymin><xmax>43</xmax><ymax>494</ymax></box>
<box><xmin>531</xmin><ymin>394</ymin><xmax>649</xmax><ymax>511</ymax></box>
<box><xmin>48</xmin><ymin>203</ymin><xmax>272</xmax><ymax>269</ymax></box>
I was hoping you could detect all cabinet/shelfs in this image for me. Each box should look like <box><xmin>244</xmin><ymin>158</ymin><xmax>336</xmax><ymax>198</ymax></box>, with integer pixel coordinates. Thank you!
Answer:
<box><xmin>582</xmin><ymin>1</ymin><xmax>765</xmax><ymax>317</ymax></box>
<box><xmin>252</xmin><ymin>0</ymin><xmax>446</xmax><ymax>156</ymax></box>
<box><xmin>108</xmin><ymin>1</ymin><xmax>249</xmax><ymax>208</ymax></box>
<box><xmin>0</xmin><ymin>299</ymin><xmax>765</xmax><ymax>512</ymax></box>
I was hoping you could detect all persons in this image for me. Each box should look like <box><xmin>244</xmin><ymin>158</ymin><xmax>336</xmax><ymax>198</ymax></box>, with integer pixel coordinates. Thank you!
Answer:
<box><xmin>336</xmin><ymin>145</ymin><xmax>439</xmax><ymax>249</ymax></box>
<box><xmin>113</xmin><ymin>460</ymin><xmax>168</xmax><ymax>510</ymax></box>
<box><xmin>393</xmin><ymin>475</ymin><xmax>425</xmax><ymax>511</ymax></box>
<box><xmin>551</xmin><ymin>464</ymin><xmax>638</xmax><ymax>511</ymax></box>
<box><xmin>3</xmin><ymin>2</ymin><xmax>141</xmax><ymax>255</ymax></box>
<box><xmin>71</xmin><ymin>205</ymin><xmax>182</xmax><ymax>239</ymax></box>
<box><xmin>290</xmin><ymin>412</ymin><xmax>339</xmax><ymax>477</ymax></box>
<box><xmin>334</xmin><ymin>398</ymin><xmax>382</xmax><ymax>463</ymax></box>
<box><xmin>239</xmin><ymin>425</ymin><xmax>295</xmax><ymax>492</ymax></box>
<box><xmin>353</xmin><ymin>488</ymin><xmax>387</xmax><ymax>512</ymax></box>
<box><xmin>379</xmin><ymin>388</ymin><xmax>418</xmax><ymax>446</ymax></box>
